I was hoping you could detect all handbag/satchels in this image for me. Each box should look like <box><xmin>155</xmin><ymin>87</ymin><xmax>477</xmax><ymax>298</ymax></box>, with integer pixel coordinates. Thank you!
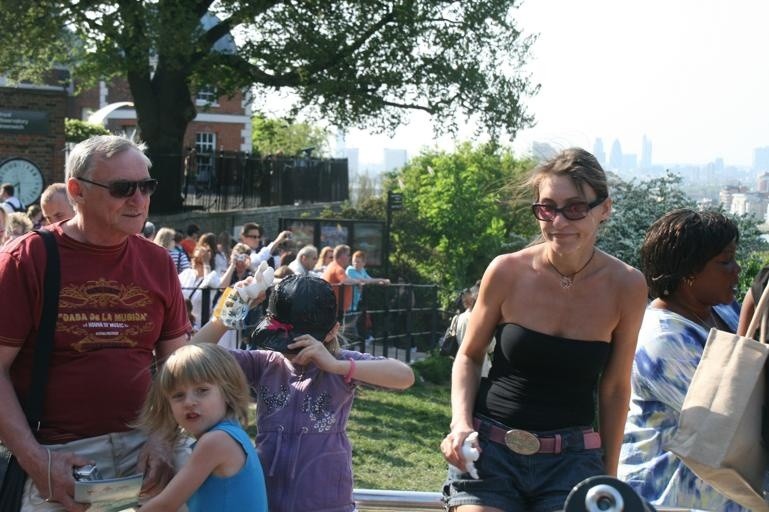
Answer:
<box><xmin>663</xmin><ymin>327</ymin><xmax>768</xmax><ymax>512</ymax></box>
<box><xmin>1</xmin><ymin>441</ymin><xmax>28</xmax><ymax>511</ymax></box>
<box><xmin>440</xmin><ymin>333</ymin><xmax>457</xmax><ymax>357</ymax></box>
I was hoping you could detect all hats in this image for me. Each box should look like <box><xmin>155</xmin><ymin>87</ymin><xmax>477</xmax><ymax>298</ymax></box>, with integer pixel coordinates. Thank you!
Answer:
<box><xmin>251</xmin><ymin>275</ymin><xmax>337</xmax><ymax>354</ymax></box>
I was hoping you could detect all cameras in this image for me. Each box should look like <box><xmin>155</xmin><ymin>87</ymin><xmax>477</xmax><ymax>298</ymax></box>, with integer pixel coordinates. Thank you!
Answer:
<box><xmin>284</xmin><ymin>232</ymin><xmax>292</xmax><ymax>239</ymax></box>
<box><xmin>238</xmin><ymin>255</ymin><xmax>244</xmax><ymax>260</ymax></box>
<box><xmin>71</xmin><ymin>464</ymin><xmax>102</xmax><ymax>482</ymax></box>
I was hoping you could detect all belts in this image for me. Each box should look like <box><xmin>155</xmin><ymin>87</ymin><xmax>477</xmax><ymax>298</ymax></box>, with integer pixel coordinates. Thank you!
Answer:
<box><xmin>472</xmin><ymin>418</ymin><xmax>601</xmax><ymax>456</ymax></box>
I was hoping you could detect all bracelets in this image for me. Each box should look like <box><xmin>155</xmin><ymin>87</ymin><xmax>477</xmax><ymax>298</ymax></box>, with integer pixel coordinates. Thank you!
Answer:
<box><xmin>344</xmin><ymin>358</ymin><xmax>354</xmax><ymax>383</ymax></box>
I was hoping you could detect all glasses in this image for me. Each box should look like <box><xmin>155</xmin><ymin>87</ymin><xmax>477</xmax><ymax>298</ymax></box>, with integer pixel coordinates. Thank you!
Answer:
<box><xmin>76</xmin><ymin>176</ymin><xmax>157</xmax><ymax>198</ymax></box>
<box><xmin>531</xmin><ymin>198</ymin><xmax>600</xmax><ymax>221</ymax></box>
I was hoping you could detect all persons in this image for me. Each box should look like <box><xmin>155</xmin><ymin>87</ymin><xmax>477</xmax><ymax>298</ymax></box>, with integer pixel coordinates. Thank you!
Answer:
<box><xmin>616</xmin><ymin>206</ymin><xmax>752</xmax><ymax>512</ymax></box>
<box><xmin>179</xmin><ymin>148</ymin><xmax>202</xmax><ymax>199</ymax></box>
<box><xmin>145</xmin><ymin>220</ymin><xmax>389</xmax><ymax>349</ymax></box>
<box><xmin>451</xmin><ymin>278</ymin><xmax>497</xmax><ymax>382</ymax></box>
<box><xmin>0</xmin><ymin>181</ymin><xmax>76</xmax><ymax>247</ymax></box>
<box><xmin>0</xmin><ymin>134</ymin><xmax>191</xmax><ymax>512</ymax></box>
<box><xmin>190</xmin><ymin>275</ymin><xmax>414</xmax><ymax>512</ymax></box>
<box><xmin>435</xmin><ymin>147</ymin><xmax>650</xmax><ymax>512</ymax></box>
<box><xmin>734</xmin><ymin>261</ymin><xmax>769</xmax><ymax>337</ymax></box>
<box><xmin>135</xmin><ymin>343</ymin><xmax>268</xmax><ymax>512</ymax></box>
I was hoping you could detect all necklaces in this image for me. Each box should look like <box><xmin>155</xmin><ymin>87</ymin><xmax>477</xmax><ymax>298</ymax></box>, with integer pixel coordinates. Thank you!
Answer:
<box><xmin>545</xmin><ymin>244</ymin><xmax>596</xmax><ymax>289</ymax></box>
<box><xmin>682</xmin><ymin>304</ymin><xmax>720</xmax><ymax>330</ymax></box>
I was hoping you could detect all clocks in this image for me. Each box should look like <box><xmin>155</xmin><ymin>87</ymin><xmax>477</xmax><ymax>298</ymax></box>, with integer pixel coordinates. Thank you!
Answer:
<box><xmin>0</xmin><ymin>156</ymin><xmax>45</xmax><ymax>210</ymax></box>
